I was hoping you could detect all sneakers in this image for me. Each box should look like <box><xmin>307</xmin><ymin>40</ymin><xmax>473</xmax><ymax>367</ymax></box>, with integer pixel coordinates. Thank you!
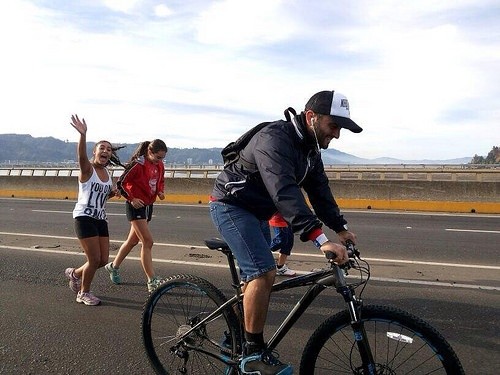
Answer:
<box><xmin>104</xmin><ymin>262</ymin><xmax>121</xmax><ymax>285</ymax></box>
<box><xmin>147</xmin><ymin>278</ymin><xmax>165</xmax><ymax>293</ymax></box>
<box><xmin>76</xmin><ymin>290</ymin><xmax>101</xmax><ymax>306</ymax></box>
<box><xmin>276</xmin><ymin>262</ymin><xmax>297</xmax><ymax>276</ymax></box>
<box><xmin>65</xmin><ymin>268</ymin><xmax>81</xmax><ymax>293</ymax></box>
<box><xmin>240</xmin><ymin>341</ymin><xmax>294</xmax><ymax>375</ymax></box>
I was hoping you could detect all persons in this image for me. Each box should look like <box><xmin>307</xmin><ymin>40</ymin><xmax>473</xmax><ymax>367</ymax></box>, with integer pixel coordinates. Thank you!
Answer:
<box><xmin>269</xmin><ymin>210</ymin><xmax>297</xmax><ymax>275</ymax></box>
<box><xmin>105</xmin><ymin>139</ymin><xmax>168</xmax><ymax>292</ymax></box>
<box><xmin>65</xmin><ymin>114</ymin><xmax>121</xmax><ymax>306</ymax></box>
<box><xmin>209</xmin><ymin>90</ymin><xmax>363</xmax><ymax>375</ymax></box>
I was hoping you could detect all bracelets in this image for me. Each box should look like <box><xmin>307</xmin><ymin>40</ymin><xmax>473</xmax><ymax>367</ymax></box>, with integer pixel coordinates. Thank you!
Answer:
<box><xmin>314</xmin><ymin>234</ymin><xmax>328</xmax><ymax>249</ymax></box>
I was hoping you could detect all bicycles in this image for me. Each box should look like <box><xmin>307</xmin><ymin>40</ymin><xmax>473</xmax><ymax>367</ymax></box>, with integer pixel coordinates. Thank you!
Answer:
<box><xmin>141</xmin><ymin>237</ymin><xmax>466</xmax><ymax>375</ymax></box>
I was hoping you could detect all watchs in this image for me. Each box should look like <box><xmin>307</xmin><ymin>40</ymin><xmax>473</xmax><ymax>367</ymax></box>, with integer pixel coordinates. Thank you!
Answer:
<box><xmin>336</xmin><ymin>225</ymin><xmax>347</xmax><ymax>234</ymax></box>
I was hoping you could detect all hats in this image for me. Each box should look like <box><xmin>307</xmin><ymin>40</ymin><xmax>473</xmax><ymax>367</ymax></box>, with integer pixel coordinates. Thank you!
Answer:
<box><xmin>304</xmin><ymin>90</ymin><xmax>363</xmax><ymax>134</ymax></box>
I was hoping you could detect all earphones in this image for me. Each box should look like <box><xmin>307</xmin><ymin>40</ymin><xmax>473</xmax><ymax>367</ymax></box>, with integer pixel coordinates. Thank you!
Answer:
<box><xmin>310</xmin><ymin>117</ymin><xmax>314</xmax><ymax>126</ymax></box>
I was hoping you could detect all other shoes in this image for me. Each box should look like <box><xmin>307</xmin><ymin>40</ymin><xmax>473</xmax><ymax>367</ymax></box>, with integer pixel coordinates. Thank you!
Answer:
<box><xmin>220</xmin><ymin>330</ymin><xmax>240</xmax><ymax>362</ymax></box>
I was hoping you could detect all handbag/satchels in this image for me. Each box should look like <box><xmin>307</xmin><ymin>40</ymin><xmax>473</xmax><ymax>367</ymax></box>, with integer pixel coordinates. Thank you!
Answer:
<box><xmin>221</xmin><ymin>120</ymin><xmax>274</xmax><ymax>169</ymax></box>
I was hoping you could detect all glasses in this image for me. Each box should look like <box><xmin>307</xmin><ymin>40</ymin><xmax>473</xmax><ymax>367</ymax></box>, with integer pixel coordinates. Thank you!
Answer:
<box><xmin>156</xmin><ymin>156</ymin><xmax>164</xmax><ymax>160</ymax></box>
